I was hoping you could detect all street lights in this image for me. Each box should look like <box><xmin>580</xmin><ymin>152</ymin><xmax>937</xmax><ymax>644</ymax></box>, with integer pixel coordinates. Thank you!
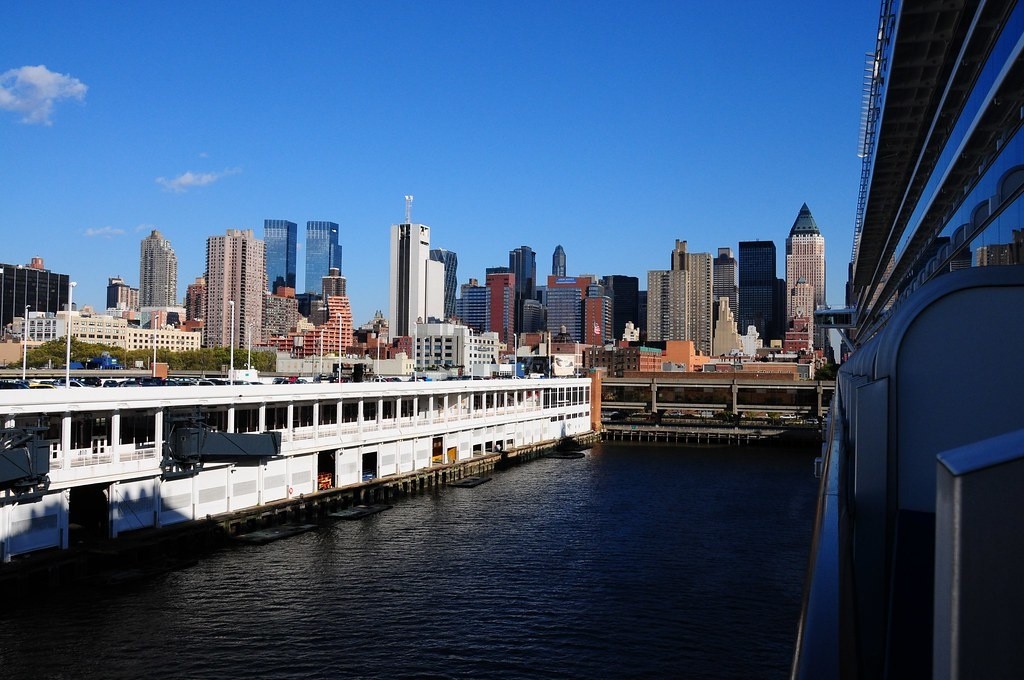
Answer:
<box><xmin>248</xmin><ymin>324</ymin><xmax>253</xmax><ymax>369</ymax></box>
<box><xmin>153</xmin><ymin>315</ymin><xmax>159</xmax><ymax>377</ymax></box>
<box><xmin>66</xmin><ymin>282</ymin><xmax>77</xmax><ymax>389</ymax></box>
<box><xmin>412</xmin><ymin>322</ymin><xmax>417</xmax><ymax>382</ymax></box>
<box><xmin>229</xmin><ymin>300</ymin><xmax>235</xmax><ymax>386</ymax></box>
<box><xmin>23</xmin><ymin>305</ymin><xmax>31</xmax><ymax>380</ymax></box>
<box><xmin>337</xmin><ymin>313</ymin><xmax>341</xmax><ymax>382</ymax></box>
<box><xmin>513</xmin><ymin>333</ymin><xmax>517</xmax><ymax>379</ymax></box>
<box><xmin>576</xmin><ymin>341</ymin><xmax>579</xmax><ymax>377</ymax></box>
<box><xmin>469</xmin><ymin>328</ymin><xmax>473</xmax><ymax>380</ymax></box>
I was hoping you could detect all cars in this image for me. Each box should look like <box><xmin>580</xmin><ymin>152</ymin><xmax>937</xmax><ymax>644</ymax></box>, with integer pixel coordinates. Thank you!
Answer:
<box><xmin>0</xmin><ymin>376</ymin><xmax>308</xmax><ymax>390</ymax></box>
<box><xmin>314</xmin><ymin>374</ymin><xmax>486</xmax><ymax>383</ymax></box>
